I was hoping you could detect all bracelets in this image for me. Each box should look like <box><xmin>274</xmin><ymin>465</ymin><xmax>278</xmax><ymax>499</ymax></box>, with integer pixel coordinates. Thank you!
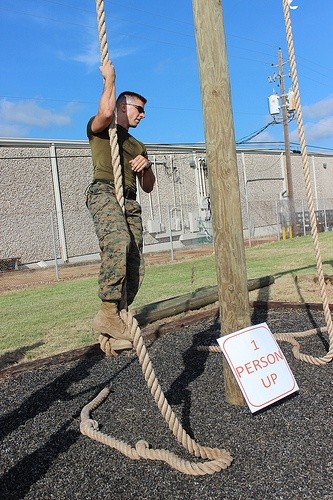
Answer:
<box><xmin>142</xmin><ymin>161</ymin><xmax>152</xmax><ymax>171</ymax></box>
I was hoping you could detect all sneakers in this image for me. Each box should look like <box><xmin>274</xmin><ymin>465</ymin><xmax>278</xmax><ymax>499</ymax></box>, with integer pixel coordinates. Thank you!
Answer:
<box><xmin>108</xmin><ymin>337</ymin><xmax>132</xmax><ymax>350</ymax></box>
<box><xmin>92</xmin><ymin>300</ymin><xmax>133</xmax><ymax>341</ymax></box>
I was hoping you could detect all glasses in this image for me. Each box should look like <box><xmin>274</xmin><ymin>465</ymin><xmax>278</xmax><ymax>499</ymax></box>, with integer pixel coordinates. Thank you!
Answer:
<box><xmin>124</xmin><ymin>103</ymin><xmax>145</xmax><ymax>114</ymax></box>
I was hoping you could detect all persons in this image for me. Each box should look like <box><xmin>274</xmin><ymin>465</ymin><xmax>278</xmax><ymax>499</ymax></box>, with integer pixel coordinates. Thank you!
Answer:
<box><xmin>86</xmin><ymin>61</ymin><xmax>155</xmax><ymax>354</ymax></box>
